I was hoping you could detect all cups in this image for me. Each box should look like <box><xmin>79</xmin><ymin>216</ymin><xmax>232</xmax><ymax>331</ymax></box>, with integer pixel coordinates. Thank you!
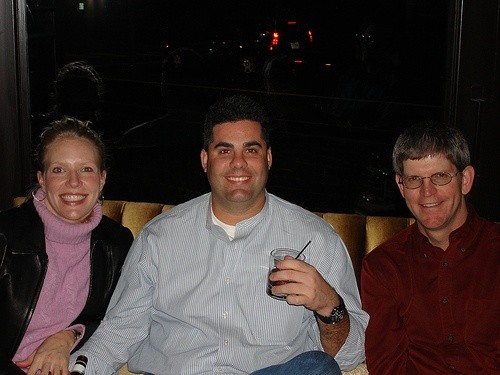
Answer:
<box><xmin>267</xmin><ymin>248</ymin><xmax>306</xmax><ymax>300</ymax></box>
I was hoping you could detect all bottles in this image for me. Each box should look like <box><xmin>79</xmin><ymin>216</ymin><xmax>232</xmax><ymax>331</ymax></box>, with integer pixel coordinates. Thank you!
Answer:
<box><xmin>70</xmin><ymin>355</ymin><xmax>88</xmax><ymax>375</ymax></box>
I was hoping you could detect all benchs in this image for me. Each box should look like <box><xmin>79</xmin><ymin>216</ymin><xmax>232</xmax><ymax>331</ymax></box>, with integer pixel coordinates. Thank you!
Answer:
<box><xmin>14</xmin><ymin>197</ymin><xmax>416</xmax><ymax>375</ymax></box>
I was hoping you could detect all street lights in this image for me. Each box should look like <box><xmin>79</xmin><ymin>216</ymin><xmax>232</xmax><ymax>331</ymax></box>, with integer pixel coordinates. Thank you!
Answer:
<box><xmin>53</xmin><ymin>0</ymin><xmax>85</xmax><ymax>75</ymax></box>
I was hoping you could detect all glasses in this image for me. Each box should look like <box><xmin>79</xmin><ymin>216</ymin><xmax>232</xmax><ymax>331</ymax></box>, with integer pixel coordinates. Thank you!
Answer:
<box><xmin>399</xmin><ymin>171</ymin><xmax>458</xmax><ymax>189</ymax></box>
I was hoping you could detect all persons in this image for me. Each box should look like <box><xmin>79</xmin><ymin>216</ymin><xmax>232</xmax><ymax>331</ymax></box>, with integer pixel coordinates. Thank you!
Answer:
<box><xmin>359</xmin><ymin>124</ymin><xmax>500</xmax><ymax>375</ymax></box>
<box><xmin>0</xmin><ymin>115</ymin><xmax>135</xmax><ymax>375</ymax></box>
<box><xmin>32</xmin><ymin>95</ymin><xmax>370</xmax><ymax>375</ymax></box>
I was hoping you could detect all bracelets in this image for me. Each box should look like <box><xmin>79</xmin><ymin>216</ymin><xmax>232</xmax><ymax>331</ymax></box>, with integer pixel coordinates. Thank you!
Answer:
<box><xmin>72</xmin><ymin>329</ymin><xmax>81</xmax><ymax>341</ymax></box>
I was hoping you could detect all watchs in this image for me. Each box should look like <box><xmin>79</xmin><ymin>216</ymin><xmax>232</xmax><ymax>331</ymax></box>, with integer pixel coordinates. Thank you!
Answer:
<box><xmin>316</xmin><ymin>295</ymin><xmax>347</xmax><ymax>325</ymax></box>
